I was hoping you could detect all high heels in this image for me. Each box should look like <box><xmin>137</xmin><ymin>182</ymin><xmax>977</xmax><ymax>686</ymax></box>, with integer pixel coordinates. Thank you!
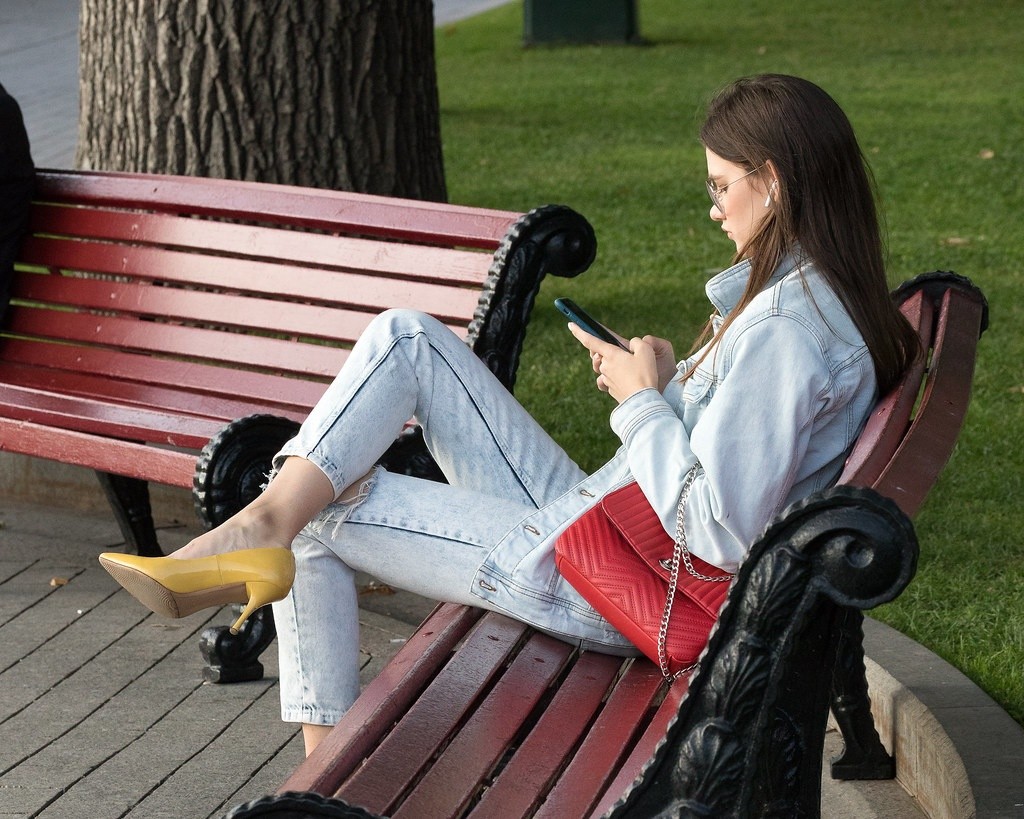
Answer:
<box><xmin>97</xmin><ymin>547</ymin><xmax>296</xmax><ymax>634</ymax></box>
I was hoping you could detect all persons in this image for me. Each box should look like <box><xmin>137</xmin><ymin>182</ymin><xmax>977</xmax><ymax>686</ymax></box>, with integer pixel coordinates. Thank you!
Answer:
<box><xmin>97</xmin><ymin>74</ymin><xmax>923</xmax><ymax>758</ymax></box>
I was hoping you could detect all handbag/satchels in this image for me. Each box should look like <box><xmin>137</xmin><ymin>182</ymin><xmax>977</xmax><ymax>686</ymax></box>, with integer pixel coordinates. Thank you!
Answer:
<box><xmin>556</xmin><ymin>481</ymin><xmax>736</xmax><ymax>681</ymax></box>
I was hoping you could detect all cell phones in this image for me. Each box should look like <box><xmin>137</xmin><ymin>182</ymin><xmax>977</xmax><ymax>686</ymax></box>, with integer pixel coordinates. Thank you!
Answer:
<box><xmin>555</xmin><ymin>297</ymin><xmax>632</xmax><ymax>354</ymax></box>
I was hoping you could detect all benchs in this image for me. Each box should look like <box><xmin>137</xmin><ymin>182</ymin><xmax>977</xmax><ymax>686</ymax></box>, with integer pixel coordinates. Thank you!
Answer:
<box><xmin>0</xmin><ymin>169</ymin><xmax>599</xmax><ymax>686</ymax></box>
<box><xmin>222</xmin><ymin>269</ymin><xmax>989</xmax><ymax>819</ymax></box>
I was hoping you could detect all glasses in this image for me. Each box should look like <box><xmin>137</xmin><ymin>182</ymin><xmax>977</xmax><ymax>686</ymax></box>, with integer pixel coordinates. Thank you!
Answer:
<box><xmin>705</xmin><ymin>162</ymin><xmax>768</xmax><ymax>217</ymax></box>
